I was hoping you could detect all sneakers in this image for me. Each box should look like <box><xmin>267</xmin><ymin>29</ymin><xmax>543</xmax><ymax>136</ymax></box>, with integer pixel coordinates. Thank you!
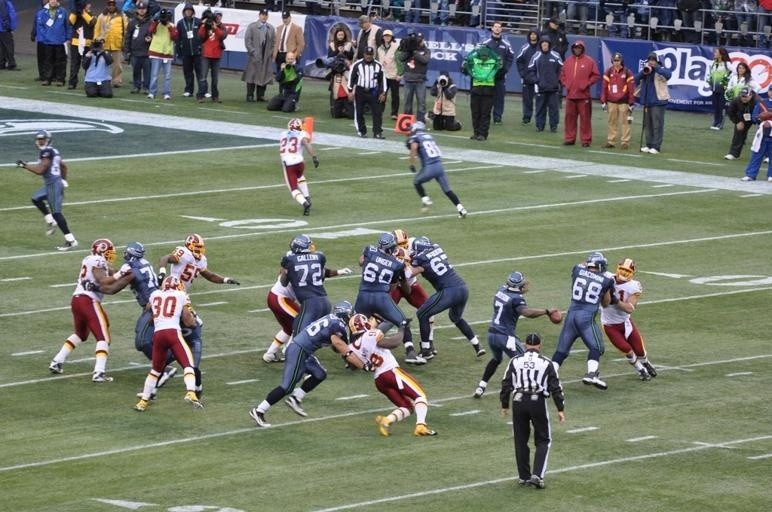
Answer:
<box><xmin>403</xmin><ymin>340</ymin><xmax>439</xmax><ymax>365</ymax></box>
<box><xmin>248</xmin><ymin>406</ymin><xmax>272</xmax><ymax>429</ymax></box>
<box><xmin>262</xmin><ymin>350</ymin><xmax>286</xmax><ymax>363</ymax></box>
<box><xmin>185</xmin><ymin>389</ymin><xmax>206</xmax><ymax>412</ymax></box>
<box><xmin>637</xmin><ymin>361</ymin><xmax>658</xmax><ymax>382</ymax></box>
<box><xmin>580</xmin><ymin>371</ymin><xmax>608</xmax><ymax>389</ymax></box>
<box><xmin>48</xmin><ymin>360</ymin><xmax>66</xmax><ymax>375</ymax></box>
<box><xmin>376</xmin><ymin>414</ymin><xmax>391</xmax><ymax>436</ymax></box>
<box><xmin>134</xmin><ymin>389</ymin><xmax>159</xmax><ymax>413</ymax></box>
<box><xmin>456</xmin><ymin>206</ymin><xmax>467</xmax><ymax>220</ymax></box>
<box><xmin>419</xmin><ymin>201</ymin><xmax>433</xmax><ymax>213</ymax></box>
<box><xmin>472</xmin><ymin>387</ymin><xmax>485</xmax><ymax>398</ymax></box>
<box><xmin>519</xmin><ymin>474</ymin><xmax>545</xmax><ymax>489</ymax></box>
<box><xmin>302</xmin><ymin>196</ymin><xmax>313</xmax><ymax>216</ymax></box>
<box><xmin>413</xmin><ymin>423</ymin><xmax>439</xmax><ymax>437</ymax></box>
<box><xmin>471</xmin><ymin>342</ymin><xmax>486</xmax><ymax>357</ymax></box>
<box><xmin>44</xmin><ymin>222</ymin><xmax>79</xmax><ymax>252</ymax></box>
<box><xmin>283</xmin><ymin>394</ymin><xmax>309</xmax><ymax>419</ymax></box>
<box><xmin>91</xmin><ymin>370</ymin><xmax>114</xmax><ymax>384</ymax></box>
<box><xmin>0</xmin><ymin>62</ymin><xmax>268</xmax><ymax>104</ymax></box>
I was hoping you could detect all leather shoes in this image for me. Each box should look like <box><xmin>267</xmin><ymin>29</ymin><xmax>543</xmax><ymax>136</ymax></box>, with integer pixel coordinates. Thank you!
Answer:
<box><xmin>359</xmin><ymin>133</ymin><xmax>387</xmax><ymax>140</ymax></box>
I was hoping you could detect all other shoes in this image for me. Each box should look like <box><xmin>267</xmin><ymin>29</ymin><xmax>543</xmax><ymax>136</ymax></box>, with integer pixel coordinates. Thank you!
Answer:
<box><xmin>723</xmin><ymin>154</ymin><xmax>740</xmax><ymax>160</ymax></box>
<box><xmin>537</xmin><ymin>127</ymin><xmax>545</xmax><ymax>132</ymax></box>
<box><xmin>641</xmin><ymin>146</ymin><xmax>650</xmax><ymax>152</ymax></box>
<box><xmin>471</xmin><ymin>133</ymin><xmax>478</xmax><ymax>140</ymax></box>
<box><xmin>741</xmin><ymin>176</ymin><xmax>754</xmax><ymax>181</ymax></box>
<box><xmin>649</xmin><ymin>148</ymin><xmax>659</xmax><ymax>154</ymax></box>
<box><xmin>476</xmin><ymin>134</ymin><xmax>486</xmax><ymax>140</ymax></box>
<box><xmin>709</xmin><ymin>125</ymin><xmax>720</xmax><ymax>130</ymax></box>
<box><xmin>621</xmin><ymin>144</ymin><xmax>628</xmax><ymax>150</ymax></box>
<box><xmin>550</xmin><ymin>126</ymin><xmax>557</xmax><ymax>131</ymax></box>
<box><xmin>602</xmin><ymin>143</ymin><xmax>614</xmax><ymax>148</ymax></box>
<box><xmin>494</xmin><ymin>121</ymin><xmax>503</xmax><ymax>125</ymax></box>
<box><xmin>764</xmin><ymin>157</ymin><xmax>770</xmax><ymax>163</ymax></box>
<box><xmin>583</xmin><ymin>142</ymin><xmax>588</xmax><ymax>147</ymax></box>
<box><xmin>563</xmin><ymin>140</ymin><xmax>576</xmax><ymax>146</ymax></box>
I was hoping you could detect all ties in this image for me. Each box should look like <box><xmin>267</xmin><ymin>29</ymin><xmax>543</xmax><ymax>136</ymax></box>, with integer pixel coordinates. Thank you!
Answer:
<box><xmin>280</xmin><ymin>26</ymin><xmax>288</xmax><ymax>52</ymax></box>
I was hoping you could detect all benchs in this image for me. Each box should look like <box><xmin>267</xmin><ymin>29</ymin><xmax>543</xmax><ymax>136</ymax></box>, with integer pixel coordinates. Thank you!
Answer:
<box><xmin>218</xmin><ymin>0</ymin><xmax>771</xmax><ymax>54</ymax></box>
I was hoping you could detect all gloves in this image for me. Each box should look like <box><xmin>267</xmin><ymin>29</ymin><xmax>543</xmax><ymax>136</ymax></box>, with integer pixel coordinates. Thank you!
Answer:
<box><xmin>15</xmin><ymin>158</ymin><xmax>29</xmax><ymax>169</ymax></box>
<box><xmin>158</xmin><ymin>272</ymin><xmax>167</xmax><ymax>286</ymax></box>
<box><xmin>363</xmin><ymin>360</ymin><xmax>377</xmax><ymax>372</ymax></box>
<box><xmin>62</xmin><ymin>178</ymin><xmax>69</xmax><ymax>189</ymax></box>
<box><xmin>336</xmin><ymin>266</ymin><xmax>354</xmax><ymax>276</ymax></box>
<box><xmin>312</xmin><ymin>154</ymin><xmax>321</xmax><ymax>170</ymax></box>
<box><xmin>408</xmin><ymin>163</ymin><xmax>417</xmax><ymax>174</ymax></box>
<box><xmin>226</xmin><ymin>276</ymin><xmax>241</xmax><ymax>286</ymax></box>
<box><xmin>80</xmin><ymin>279</ymin><xmax>101</xmax><ymax>293</ymax></box>
<box><xmin>400</xmin><ymin>316</ymin><xmax>412</xmax><ymax>328</ymax></box>
<box><xmin>113</xmin><ymin>263</ymin><xmax>132</xmax><ymax>281</ymax></box>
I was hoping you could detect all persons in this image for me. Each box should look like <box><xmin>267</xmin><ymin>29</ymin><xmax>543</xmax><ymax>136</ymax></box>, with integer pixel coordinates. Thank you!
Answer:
<box><xmin>48</xmin><ymin>233</ymin><xmax>242</xmax><ymax>413</ymax></box>
<box><xmin>707</xmin><ymin>49</ymin><xmax>772</xmax><ymax>182</ymax></box>
<box><xmin>0</xmin><ymin>0</ymin><xmax>227</xmax><ymax>103</ymax></box>
<box><xmin>280</xmin><ymin>118</ymin><xmax>320</xmax><ymax>216</ymax></box>
<box><xmin>472</xmin><ymin>251</ymin><xmax>656</xmax><ymax>398</ymax></box>
<box><xmin>249</xmin><ymin>229</ymin><xmax>485</xmax><ymax>435</ymax></box>
<box><xmin>241</xmin><ymin>8</ymin><xmax>304</xmax><ymax>112</ymax></box>
<box><xmin>517</xmin><ymin>19</ymin><xmax>672</xmax><ymax>154</ymax></box>
<box><xmin>500</xmin><ymin>335</ymin><xmax>565</xmax><ymax>488</ymax></box>
<box><xmin>264</xmin><ymin>0</ymin><xmax>496</xmax><ymax>29</ymax></box>
<box><xmin>406</xmin><ymin>121</ymin><xmax>467</xmax><ymax>220</ymax></box>
<box><xmin>461</xmin><ymin>21</ymin><xmax>514</xmax><ymax>140</ymax></box>
<box><xmin>544</xmin><ymin>1</ymin><xmax>771</xmax><ymax>50</ymax></box>
<box><xmin>17</xmin><ymin>131</ymin><xmax>78</xmax><ymax>251</ymax></box>
<box><xmin>327</xmin><ymin>15</ymin><xmax>461</xmax><ymax>138</ymax></box>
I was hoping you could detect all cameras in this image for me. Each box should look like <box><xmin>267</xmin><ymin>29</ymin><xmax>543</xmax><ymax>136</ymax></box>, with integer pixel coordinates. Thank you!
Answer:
<box><xmin>285</xmin><ymin>63</ymin><xmax>291</xmax><ymax>70</ymax></box>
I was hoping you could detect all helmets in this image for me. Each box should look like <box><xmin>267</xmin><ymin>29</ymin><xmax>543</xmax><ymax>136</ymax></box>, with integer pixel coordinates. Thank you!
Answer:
<box><xmin>377</xmin><ymin>232</ymin><xmax>397</xmax><ymax>252</ymax></box>
<box><xmin>123</xmin><ymin>241</ymin><xmax>147</xmax><ymax>262</ymax></box>
<box><xmin>161</xmin><ymin>275</ymin><xmax>185</xmax><ymax>291</ymax></box>
<box><xmin>90</xmin><ymin>238</ymin><xmax>117</xmax><ymax>264</ymax></box>
<box><xmin>185</xmin><ymin>233</ymin><xmax>205</xmax><ymax>257</ymax></box>
<box><xmin>409</xmin><ymin>121</ymin><xmax>426</xmax><ymax>134</ymax></box>
<box><xmin>348</xmin><ymin>313</ymin><xmax>370</xmax><ymax>336</ymax></box>
<box><xmin>409</xmin><ymin>235</ymin><xmax>433</xmax><ymax>258</ymax></box>
<box><xmin>506</xmin><ymin>271</ymin><xmax>527</xmax><ymax>288</ymax></box>
<box><xmin>332</xmin><ymin>299</ymin><xmax>354</xmax><ymax>322</ymax></box>
<box><xmin>615</xmin><ymin>258</ymin><xmax>637</xmax><ymax>283</ymax></box>
<box><xmin>586</xmin><ymin>250</ymin><xmax>608</xmax><ymax>268</ymax></box>
<box><xmin>35</xmin><ymin>129</ymin><xmax>53</xmax><ymax>150</ymax></box>
<box><xmin>287</xmin><ymin>117</ymin><xmax>303</xmax><ymax>132</ymax></box>
<box><xmin>392</xmin><ymin>229</ymin><xmax>409</xmax><ymax>250</ymax></box>
<box><xmin>290</xmin><ymin>234</ymin><xmax>313</xmax><ymax>254</ymax></box>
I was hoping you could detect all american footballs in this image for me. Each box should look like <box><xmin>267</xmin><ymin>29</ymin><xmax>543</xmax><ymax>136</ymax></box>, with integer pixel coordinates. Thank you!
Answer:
<box><xmin>550</xmin><ymin>310</ymin><xmax>563</xmax><ymax>323</ymax></box>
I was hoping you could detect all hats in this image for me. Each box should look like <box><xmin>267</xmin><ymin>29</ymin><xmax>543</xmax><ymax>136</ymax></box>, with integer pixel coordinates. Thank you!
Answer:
<box><xmin>647</xmin><ymin>53</ymin><xmax>658</xmax><ymax>62</ymax></box>
<box><xmin>281</xmin><ymin>9</ymin><xmax>291</xmax><ymax>19</ymax></box>
<box><xmin>383</xmin><ymin>30</ymin><xmax>393</xmax><ymax>36</ymax></box>
<box><xmin>137</xmin><ymin>2</ymin><xmax>148</xmax><ymax>10</ymax></box>
<box><xmin>524</xmin><ymin>332</ymin><xmax>542</xmax><ymax>345</ymax></box>
<box><xmin>739</xmin><ymin>86</ymin><xmax>751</xmax><ymax>97</ymax></box>
<box><xmin>549</xmin><ymin>17</ymin><xmax>560</xmax><ymax>27</ymax></box>
<box><xmin>363</xmin><ymin>47</ymin><xmax>374</xmax><ymax>55</ymax></box>
<box><xmin>260</xmin><ymin>8</ymin><xmax>268</xmax><ymax>13</ymax></box>
<box><xmin>611</xmin><ymin>53</ymin><xmax>623</xmax><ymax>62</ymax></box>
